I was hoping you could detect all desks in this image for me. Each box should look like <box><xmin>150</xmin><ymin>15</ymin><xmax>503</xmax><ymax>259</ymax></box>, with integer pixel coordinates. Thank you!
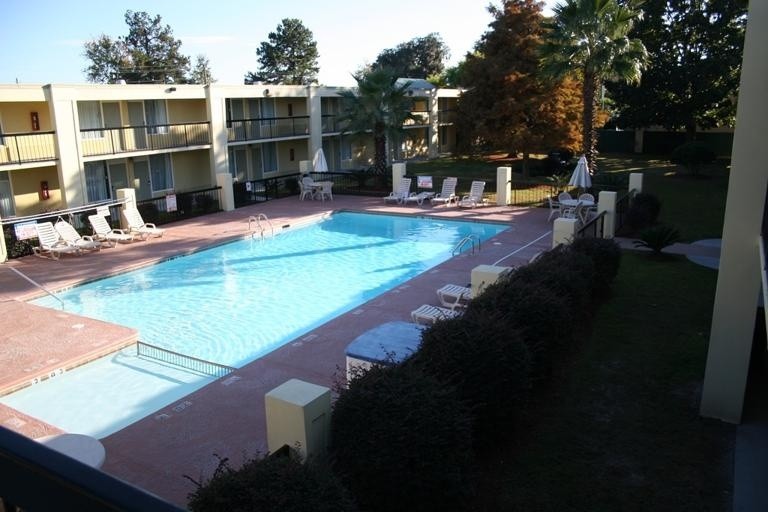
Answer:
<box><xmin>31</xmin><ymin>433</ymin><xmax>106</xmax><ymax>468</ymax></box>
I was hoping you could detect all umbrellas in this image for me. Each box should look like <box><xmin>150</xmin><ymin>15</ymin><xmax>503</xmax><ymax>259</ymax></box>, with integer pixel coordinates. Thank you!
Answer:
<box><xmin>312</xmin><ymin>147</ymin><xmax>328</xmax><ymax>180</ymax></box>
<box><xmin>567</xmin><ymin>153</ymin><xmax>592</xmax><ymax>202</ymax></box>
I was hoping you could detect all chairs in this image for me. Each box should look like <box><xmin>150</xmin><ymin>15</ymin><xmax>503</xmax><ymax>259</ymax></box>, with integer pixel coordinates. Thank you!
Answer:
<box><xmin>429</xmin><ymin>178</ymin><xmax>459</xmax><ymax>207</ymax></box>
<box><xmin>546</xmin><ymin>190</ymin><xmax>595</xmax><ymax>226</ymax></box>
<box><xmin>87</xmin><ymin>213</ymin><xmax>133</xmax><ymax>248</ymax></box>
<box><xmin>403</xmin><ymin>189</ymin><xmax>435</xmax><ymax>206</ymax></box>
<box><xmin>123</xmin><ymin>211</ymin><xmax>166</xmax><ymax>240</ymax></box>
<box><xmin>298</xmin><ymin>177</ymin><xmax>334</xmax><ymax>203</ymax></box>
<box><xmin>381</xmin><ymin>177</ymin><xmax>411</xmax><ymax>204</ymax></box>
<box><xmin>31</xmin><ymin>222</ymin><xmax>77</xmax><ymax>260</ymax></box>
<box><xmin>56</xmin><ymin>222</ymin><xmax>100</xmax><ymax>256</ymax></box>
<box><xmin>461</xmin><ymin>180</ymin><xmax>485</xmax><ymax>210</ymax></box>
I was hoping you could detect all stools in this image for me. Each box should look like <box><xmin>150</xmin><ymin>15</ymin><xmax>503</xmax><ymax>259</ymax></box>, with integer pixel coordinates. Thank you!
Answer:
<box><xmin>408</xmin><ymin>302</ymin><xmax>456</xmax><ymax>326</ymax></box>
<box><xmin>435</xmin><ymin>283</ymin><xmax>471</xmax><ymax>308</ymax></box>
